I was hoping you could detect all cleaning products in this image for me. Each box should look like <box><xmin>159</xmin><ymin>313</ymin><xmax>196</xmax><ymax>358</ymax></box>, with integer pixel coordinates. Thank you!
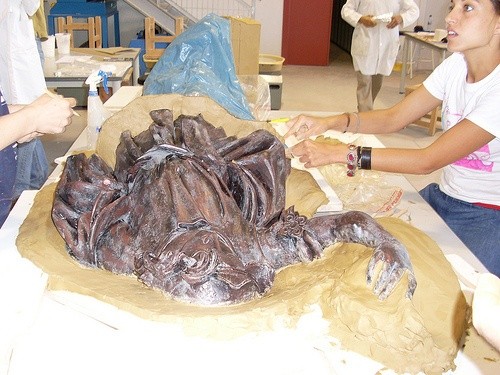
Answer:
<box><xmin>85</xmin><ymin>69</ymin><xmax>113</xmax><ymax>148</ymax></box>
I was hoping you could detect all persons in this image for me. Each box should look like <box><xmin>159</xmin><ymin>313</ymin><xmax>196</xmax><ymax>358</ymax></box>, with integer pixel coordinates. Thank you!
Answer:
<box><xmin>0</xmin><ymin>0</ymin><xmax>76</xmax><ymax>230</ymax></box>
<box><xmin>51</xmin><ymin>108</ymin><xmax>417</xmax><ymax>306</ymax></box>
<box><xmin>282</xmin><ymin>0</ymin><xmax>500</xmax><ymax>278</ymax></box>
<box><xmin>341</xmin><ymin>0</ymin><xmax>420</xmax><ymax>112</ymax></box>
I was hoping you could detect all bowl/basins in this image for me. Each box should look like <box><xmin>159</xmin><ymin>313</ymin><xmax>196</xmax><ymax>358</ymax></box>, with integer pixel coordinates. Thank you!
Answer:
<box><xmin>259</xmin><ymin>54</ymin><xmax>285</xmax><ymax>72</ymax></box>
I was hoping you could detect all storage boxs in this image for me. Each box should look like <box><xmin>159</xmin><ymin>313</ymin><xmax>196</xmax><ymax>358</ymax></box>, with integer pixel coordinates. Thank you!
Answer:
<box><xmin>227</xmin><ymin>15</ymin><xmax>261</xmax><ymax>105</ymax></box>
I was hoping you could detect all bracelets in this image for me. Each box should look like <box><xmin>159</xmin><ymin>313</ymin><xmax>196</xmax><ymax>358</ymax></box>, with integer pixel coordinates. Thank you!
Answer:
<box><xmin>361</xmin><ymin>147</ymin><xmax>372</xmax><ymax>170</ymax></box>
<box><xmin>347</xmin><ymin>143</ymin><xmax>356</xmax><ymax>177</ymax></box>
<box><xmin>357</xmin><ymin>146</ymin><xmax>361</xmax><ymax>170</ymax></box>
<box><xmin>352</xmin><ymin>112</ymin><xmax>360</xmax><ymax>134</ymax></box>
<box><xmin>342</xmin><ymin>112</ymin><xmax>351</xmax><ymax>133</ymax></box>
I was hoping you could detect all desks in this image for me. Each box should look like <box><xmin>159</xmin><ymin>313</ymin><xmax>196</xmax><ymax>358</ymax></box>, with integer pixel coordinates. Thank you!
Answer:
<box><xmin>399</xmin><ymin>30</ymin><xmax>452</xmax><ymax>94</ymax></box>
<box><xmin>41</xmin><ymin>46</ymin><xmax>142</xmax><ymax>102</ymax></box>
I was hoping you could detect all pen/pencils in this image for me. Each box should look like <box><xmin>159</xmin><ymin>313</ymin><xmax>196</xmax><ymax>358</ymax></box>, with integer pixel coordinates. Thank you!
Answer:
<box><xmin>44</xmin><ymin>89</ymin><xmax>80</xmax><ymax>118</ymax></box>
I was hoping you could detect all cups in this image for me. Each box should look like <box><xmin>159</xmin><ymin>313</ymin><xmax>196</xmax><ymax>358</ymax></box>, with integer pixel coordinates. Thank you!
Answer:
<box><xmin>55</xmin><ymin>33</ymin><xmax>71</xmax><ymax>54</ymax></box>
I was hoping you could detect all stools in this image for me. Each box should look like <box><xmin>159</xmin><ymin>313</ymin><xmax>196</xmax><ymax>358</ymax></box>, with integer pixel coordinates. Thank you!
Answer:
<box><xmin>405</xmin><ymin>83</ymin><xmax>445</xmax><ymax>135</ymax></box>
<box><xmin>55</xmin><ymin>15</ymin><xmax>102</xmax><ymax>49</ymax></box>
<box><xmin>142</xmin><ymin>16</ymin><xmax>184</xmax><ymax>70</ymax></box>
